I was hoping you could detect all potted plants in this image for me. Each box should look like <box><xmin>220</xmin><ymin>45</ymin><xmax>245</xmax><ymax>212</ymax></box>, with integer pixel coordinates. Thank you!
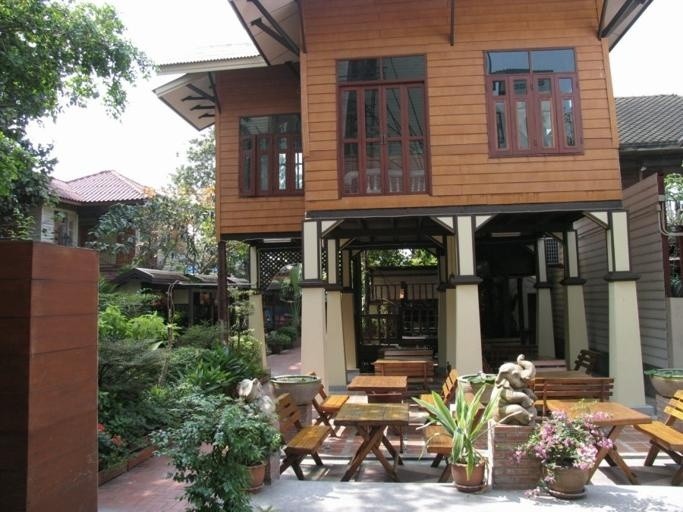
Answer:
<box><xmin>205</xmin><ymin>394</ymin><xmax>281</xmax><ymax>493</ymax></box>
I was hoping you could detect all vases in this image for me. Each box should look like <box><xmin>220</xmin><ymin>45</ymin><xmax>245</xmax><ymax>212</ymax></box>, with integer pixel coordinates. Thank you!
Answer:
<box><xmin>541</xmin><ymin>462</ymin><xmax>589</xmax><ymax>494</ymax></box>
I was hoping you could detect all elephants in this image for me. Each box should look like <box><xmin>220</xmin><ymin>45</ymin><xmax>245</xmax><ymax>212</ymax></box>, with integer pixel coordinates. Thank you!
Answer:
<box><xmin>489</xmin><ymin>353</ymin><xmax>538</xmax><ymax>428</ymax></box>
